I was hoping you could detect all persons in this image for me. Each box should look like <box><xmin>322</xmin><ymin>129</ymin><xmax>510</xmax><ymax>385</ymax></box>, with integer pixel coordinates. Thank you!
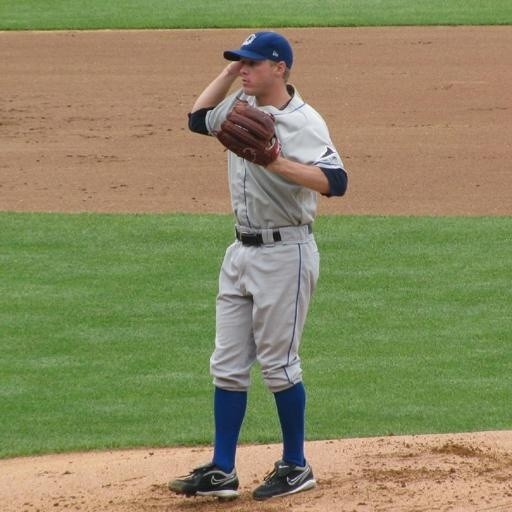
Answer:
<box><xmin>165</xmin><ymin>30</ymin><xmax>349</xmax><ymax>500</ymax></box>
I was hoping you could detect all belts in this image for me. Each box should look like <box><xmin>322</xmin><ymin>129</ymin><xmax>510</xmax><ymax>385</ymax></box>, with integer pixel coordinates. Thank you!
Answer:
<box><xmin>235</xmin><ymin>223</ymin><xmax>313</xmax><ymax>245</ymax></box>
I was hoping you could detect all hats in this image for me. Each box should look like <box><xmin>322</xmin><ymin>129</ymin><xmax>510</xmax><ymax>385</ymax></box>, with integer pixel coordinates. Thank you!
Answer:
<box><xmin>224</xmin><ymin>31</ymin><xmax>293</xmax><ymax>70</ymax></box>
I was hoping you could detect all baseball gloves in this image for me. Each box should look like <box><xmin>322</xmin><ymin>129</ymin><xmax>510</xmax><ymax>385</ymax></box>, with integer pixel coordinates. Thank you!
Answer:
<box><xmin>216</xmin><ymin>96</ymin><xmax>282</xmax><ymax>165</ymax></box>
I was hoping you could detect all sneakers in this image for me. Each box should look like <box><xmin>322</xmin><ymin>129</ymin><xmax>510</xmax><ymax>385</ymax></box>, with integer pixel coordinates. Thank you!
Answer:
<box><xmin>252</xmin><ymin>456</ymin><xmax>317</xmax><ymax>500</ymax></box>
<box><xmin>168</xmin><ymin>462</ymin><xmax>239</xmax><ymax>501</ymax></box>
